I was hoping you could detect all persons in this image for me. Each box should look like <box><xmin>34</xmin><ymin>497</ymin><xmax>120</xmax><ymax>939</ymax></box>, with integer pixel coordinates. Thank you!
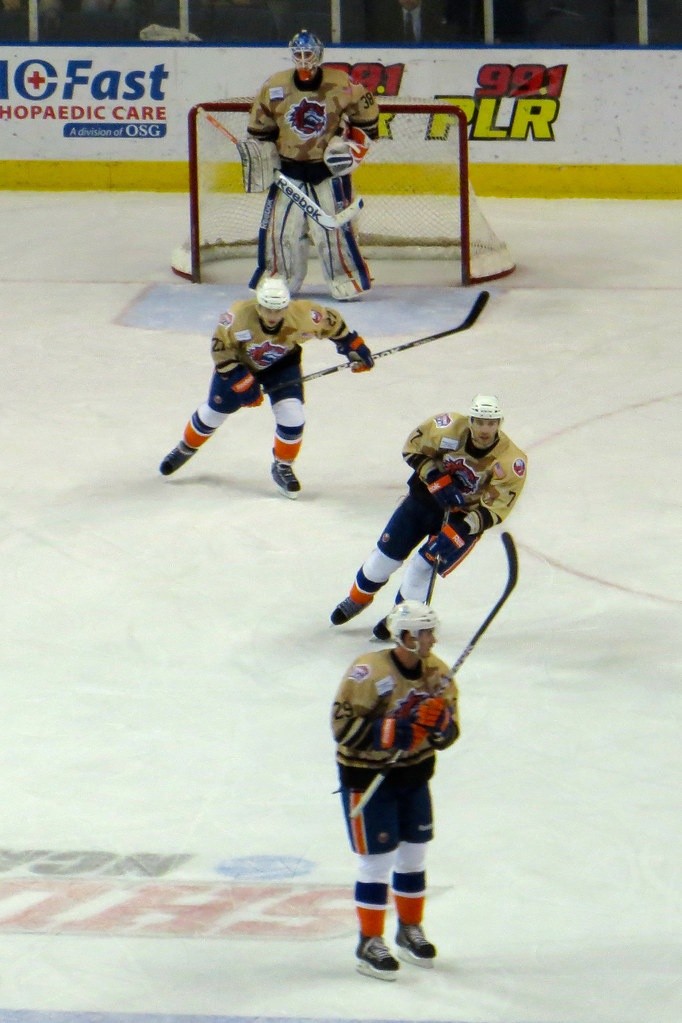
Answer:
<box><xmin>332</xmin><ymin>600</ymin><xmax>462</xmax><ymax>982</ymax></box>
<box><xmin>237</xmin><ymin>30</ymin><xmax>380</xmax><ymax>299</ymax></box>
<box><xmin>383</xmin><ymin>0</ymin><xmax>448</xmax><ymax>41</ymax></box>
<box><xmin>160</xmin><ymin>279</ymin><xmax>375</xmax><ymax>499</ymax></box>
<box><xmin>330</xmin><ymin>391</ymin><xmax>527</xmax><ymax>642</ymax></box>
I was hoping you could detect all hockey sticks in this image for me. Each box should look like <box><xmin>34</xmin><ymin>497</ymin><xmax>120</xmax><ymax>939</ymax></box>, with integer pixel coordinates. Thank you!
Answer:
<box><xmin>197</xmin><ymin>104</ymin><xmax>367</xmax><ymax>232</ymax></box>
<box><xmin>426</xmin><ymin>507</ymin><xmax>453</xmax><ymax>606</ymax></box>
<box><xmin>262</xmin><ymin>289</ymin><xmax>490</xmax><ymax>394</ymax></box>
<box><xmin>349</xmin><ymin>529</ymin><xmax>521</xmax><ymax>823</ymax></box>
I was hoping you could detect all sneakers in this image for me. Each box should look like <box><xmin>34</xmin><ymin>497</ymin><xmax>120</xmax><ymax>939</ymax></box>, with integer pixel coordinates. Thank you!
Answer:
<box><xmin>160</xmin><ymin>441</ymin><xmax>199</xmax><ymax>477</ymax></box>
<box><xmin>396</xmin><ymin>919</ymin><xmax>436</xmax><ymax>968</ymax></box>
<box><xmin>329</xmin><ymin>597</ymin><xmax>372</xmax><ymax>627</ymax></box>
<box><xmin>370</xmin><ymin>616</ymin><xmax>392</xmax><ymax>643</ymax></box>
<box><xmin>355</xmin><ymin>932</ymin><xmax>399</xmax><ymax>981</ymax></box>
<box><xmin>271</xmin><ymin>447</ymin><xmax>300</xmax><ymax>500</ymax></box>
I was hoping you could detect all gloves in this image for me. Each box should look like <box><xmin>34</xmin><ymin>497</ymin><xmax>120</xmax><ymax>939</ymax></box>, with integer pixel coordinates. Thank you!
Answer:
<box><xmin>423</xmin><ymin>469</ymin><xmax>462</xmax><ymax>510</ymax></box>
<box><xmin>337</xmin><ymin>328</ymin><xmax>374</xmax><ymax>374</ymax></box>
<box><xmin>428</xmin><ymin>522</ymin><xmax>472</xmax><ymax>559</ymax></box>
<box><xmin>416</xmin><ymin>697</ymin><xmax>452</xmax><ymax>741</ymax></box>
<box><xmin>227</xmin><ymin>367</ymin><xmax>263</xmax><ymax>408</ymax></box>
<box><xmin>379</xmin><ymin>712</ymin><xmax>427</xmax><ymax>750</ymax></box>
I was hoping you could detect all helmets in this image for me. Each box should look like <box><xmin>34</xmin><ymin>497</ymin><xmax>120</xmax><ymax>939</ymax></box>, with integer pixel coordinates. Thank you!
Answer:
<box><xmin>385</xmin><ymin>600</ymin><xmax>437</xmax><ymax>653</ymax></box>
<box><xmin>469</xmin><ymin>394</ymin><xmax>503</xmax><ymax>427</ymax></box>
<box><xmin>290</xmin><ymin>28</ymin><xmax>324</xmax><ymax>80</ymax></box>
<box><xmin>257</xmin><ymin>278</ymin><xmax>290</xmax><ymax>311</ymax></box>
